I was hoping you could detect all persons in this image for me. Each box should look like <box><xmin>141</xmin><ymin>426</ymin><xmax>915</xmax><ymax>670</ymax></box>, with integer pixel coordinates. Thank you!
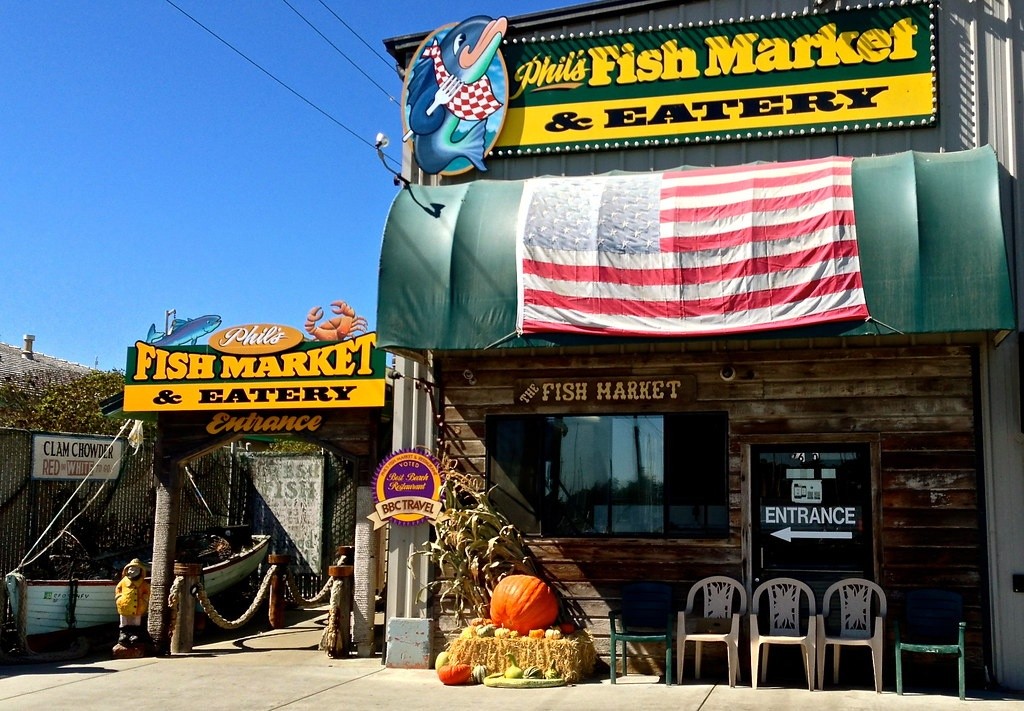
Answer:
<box><xmin>115</xmin><ymin>558</ymin><xmax>150</xmax><ymax>642</ymax></box>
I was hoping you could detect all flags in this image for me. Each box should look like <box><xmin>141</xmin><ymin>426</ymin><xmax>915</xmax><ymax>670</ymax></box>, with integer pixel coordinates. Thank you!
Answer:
<box><xmin>515</xmin><ymin>155</ymin><xmax>870</xmax><ymax>337</ymax></box>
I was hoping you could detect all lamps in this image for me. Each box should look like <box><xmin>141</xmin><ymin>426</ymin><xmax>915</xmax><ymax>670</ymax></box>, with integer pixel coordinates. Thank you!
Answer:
<box><xmin>720</xmin><ymin>339</ymin><xmax>736</xmax><ymax>381</ymax></box>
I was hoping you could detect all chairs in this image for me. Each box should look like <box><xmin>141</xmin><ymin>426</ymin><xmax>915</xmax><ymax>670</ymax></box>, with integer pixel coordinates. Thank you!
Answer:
<box><xmin>609</xmin><ymin>581</ymin><xmax>675</xmax><ymax>684</ymax></box>
<box><xmin>676</xmin><ymin>575</ymin><xmax>749</xmax><ymax>685</ymax></box>
<box><xmin>749</xmin><ymin>577</ymin><xmax>816</xmax><ymax>689</ymax></box>
<box><xmin>815</xmin><ymin>578</ymin><xmax>888</xmax><ymax>690</ymax></box>
<box><xmin>893</xmin><ymin>588</ymin><xmax>968</xmax><ymax>697</ymax></box>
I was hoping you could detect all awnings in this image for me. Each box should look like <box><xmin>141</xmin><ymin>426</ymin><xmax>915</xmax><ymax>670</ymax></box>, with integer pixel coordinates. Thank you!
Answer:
<box><xmin>374</xmin><ymin>145</ymin><xmax>1018</xmax><ymax>351</ymax></box>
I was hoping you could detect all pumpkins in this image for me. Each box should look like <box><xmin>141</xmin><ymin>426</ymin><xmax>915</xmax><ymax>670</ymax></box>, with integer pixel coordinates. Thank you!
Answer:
<box><xmin>472</xmin><ymin>574</ymin><xmax>574</xmax><ymax>640</ymax></box>
<box><xmin>435</xmin><ymin>650</ymin><xmax>557</xmax><ymax>685</ymax></box>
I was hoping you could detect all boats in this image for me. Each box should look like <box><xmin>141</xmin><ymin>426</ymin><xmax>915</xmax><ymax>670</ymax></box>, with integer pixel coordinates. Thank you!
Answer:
<box><xmin>10</xmin><ymin>531</ymin><xmax>271</xmax><ymax>653</ymax></box>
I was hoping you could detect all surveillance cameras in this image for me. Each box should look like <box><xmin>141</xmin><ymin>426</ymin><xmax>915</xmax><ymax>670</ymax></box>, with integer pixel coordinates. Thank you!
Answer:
<box><xmin>720</xmin><ymin>366</ymin><xmax>736</xmax><ymax>381</ymax></box>
